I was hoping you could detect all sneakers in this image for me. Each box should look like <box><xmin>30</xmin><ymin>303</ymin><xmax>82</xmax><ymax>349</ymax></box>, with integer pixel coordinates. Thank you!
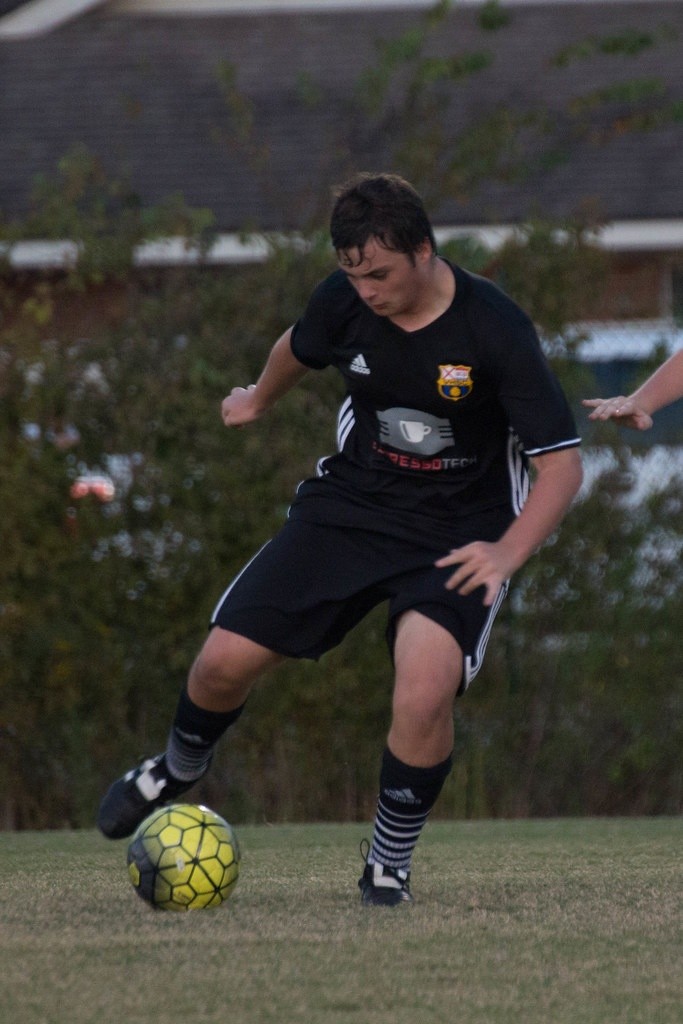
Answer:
<box><xmin>96</xmin><ymin>749</ymin><xmax>213</xmax><ymax>838</ymax></box>
<box><xmin>357</xmin><ymin>837</ymin><xmax>415</xmax><ymax>910</ymax></box>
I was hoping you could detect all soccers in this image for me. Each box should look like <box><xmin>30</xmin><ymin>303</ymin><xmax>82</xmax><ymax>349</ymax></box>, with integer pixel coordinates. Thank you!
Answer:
<box><xmin>127</xmin><ymin>800</ymin><xmax>244</xmax><ymax>915</ymax></box>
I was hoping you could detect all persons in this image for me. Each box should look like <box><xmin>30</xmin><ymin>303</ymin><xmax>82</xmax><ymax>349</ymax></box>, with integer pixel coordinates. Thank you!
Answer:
<box><xmin>580</xmin><ymin>344</ymin><xmax>683</xmax><ymax>429</ymax></box>
<box><xmin>97</xmin><ymin>174</ymin><xmax>584</xmax><ymax>906</ymax></box>
